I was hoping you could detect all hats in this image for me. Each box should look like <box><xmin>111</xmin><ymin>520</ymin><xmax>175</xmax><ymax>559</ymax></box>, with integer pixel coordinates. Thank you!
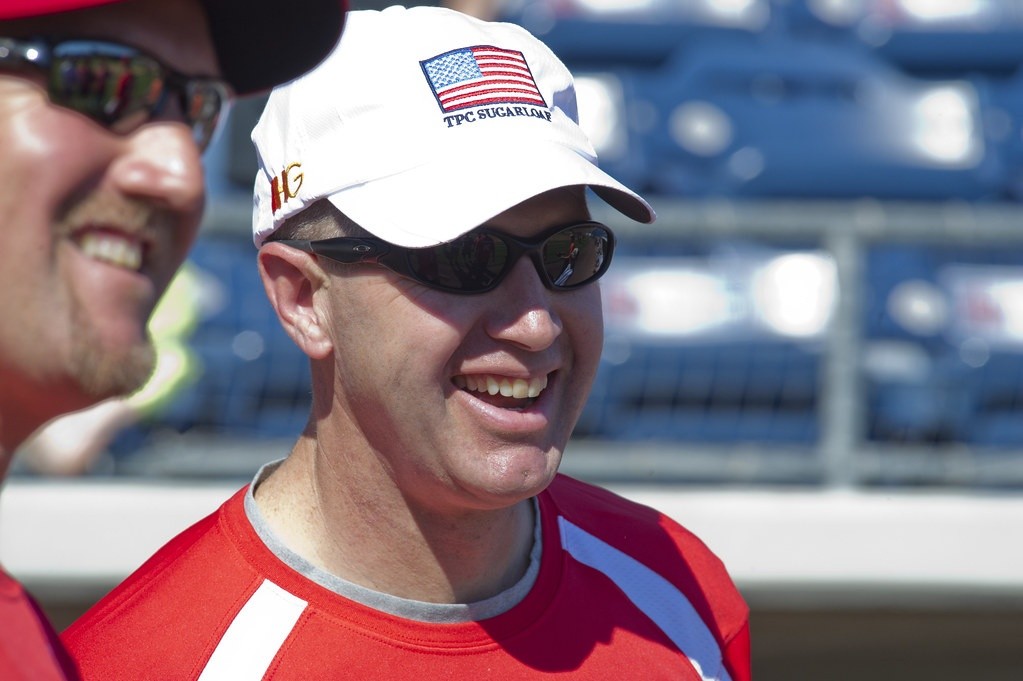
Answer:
<box><xmin>244</xmin><ymin>4</ymin><xmax>657</xmax><ymax>229</ymax></box>
<box><xmin>0</xmin><ymin>0</ymin><xmax>345</xmax><ymax>96</ymax></box>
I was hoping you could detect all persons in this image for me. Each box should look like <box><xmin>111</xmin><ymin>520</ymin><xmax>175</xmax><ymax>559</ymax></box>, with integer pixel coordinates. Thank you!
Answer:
<box><xmin>560</xmin><ymin>233</ymin><xmax>592</xmax><ymax>270</ymax></box>
<box><xmin>57</xmin><ymin>5</ymin><xmax>750</xmax><ymax>681</ymax></box>
<box><xmin>0</xmin><ymin>0</ymin><xmax>348</xmax><ymax>680</ymax></box>
<box><xmin>57</xmin><ymin>51</ymin><xmax>166</xmax><ymax>132</ymax></box>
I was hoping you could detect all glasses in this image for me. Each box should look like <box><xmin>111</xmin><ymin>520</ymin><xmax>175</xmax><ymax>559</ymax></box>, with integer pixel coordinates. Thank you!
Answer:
<box><xmin>266</xmin><ymin>220</ymin><xmax>618</xmax><ymax>293</ymax></box>
<box><xmin>0</xmin><ymin>36</ymin><xmax>237</xmax><ymax>162</ymax></box>
